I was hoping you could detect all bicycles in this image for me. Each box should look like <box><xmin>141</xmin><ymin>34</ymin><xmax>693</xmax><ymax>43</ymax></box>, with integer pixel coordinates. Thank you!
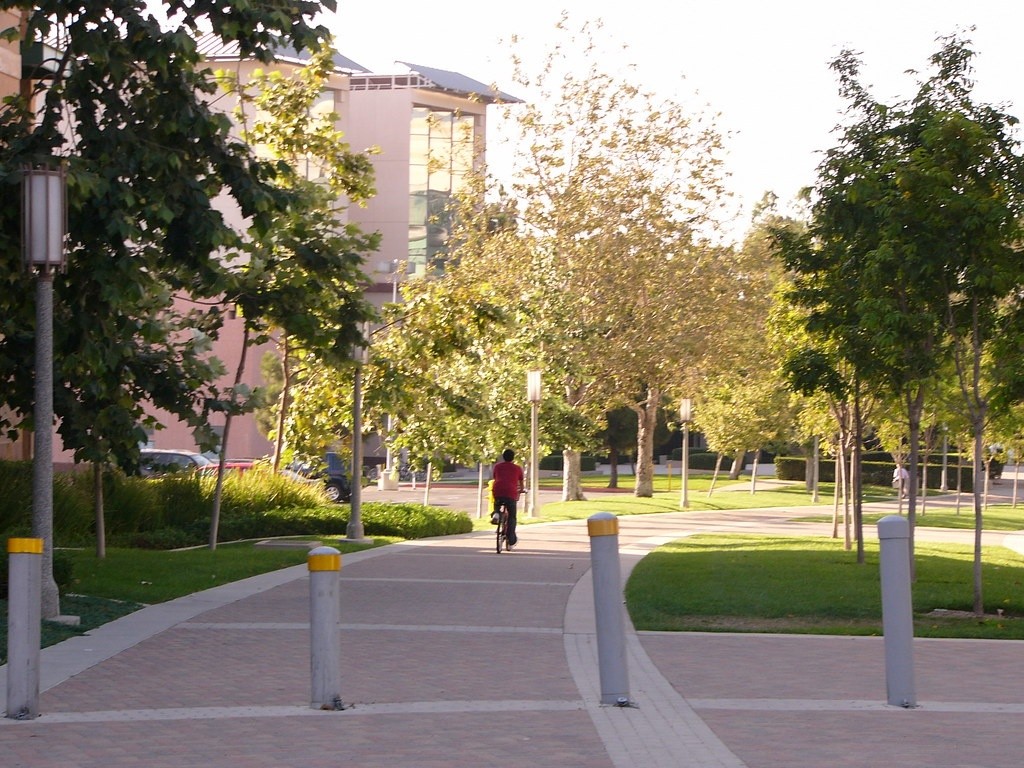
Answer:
<box><xmin>490</xmin><ymin>488</ymin><xmax>529</xmax><ymax>555</ymax></box>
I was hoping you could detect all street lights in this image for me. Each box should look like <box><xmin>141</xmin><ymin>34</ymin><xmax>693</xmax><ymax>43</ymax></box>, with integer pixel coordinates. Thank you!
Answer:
<box><xmin>526</xmin><ymin>369</ymin><xmax>540</xmax><ymax>519</ymax></box>
<box><xmin>680</xmin><ymin>398</ymin><xmax>691</xmax><ymax>508</ymax></box>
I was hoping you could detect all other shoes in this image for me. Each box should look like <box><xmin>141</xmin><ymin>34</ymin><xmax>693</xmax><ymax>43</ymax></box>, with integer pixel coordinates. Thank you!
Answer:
<box><xmin>508</xmin><ymin>539</ymin><xmax>518</xmax><ymax>549</ymax></box>
<box><xmin>492</xmin><ymin>513</ymin><xmax>499</xmax><ymax>523</ymax></box>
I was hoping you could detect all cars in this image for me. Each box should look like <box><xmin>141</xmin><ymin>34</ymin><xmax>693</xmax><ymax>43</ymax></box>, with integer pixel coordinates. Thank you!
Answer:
<box><xmin>135</xmin><ymin>448</ymin><xmax>352</xmax><ymax>504</ymax></box>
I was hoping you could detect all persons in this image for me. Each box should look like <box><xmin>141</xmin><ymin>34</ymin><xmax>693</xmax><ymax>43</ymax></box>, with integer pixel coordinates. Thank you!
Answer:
<box><xmin>491</xmin><ymin>449</ymin><xmax>525</xmax><ymax>550</ymax></box>
<box><xmin>893</xmin><ymin>465</ymin><xmax>909</xmax><ymax>499</ymax></box>
